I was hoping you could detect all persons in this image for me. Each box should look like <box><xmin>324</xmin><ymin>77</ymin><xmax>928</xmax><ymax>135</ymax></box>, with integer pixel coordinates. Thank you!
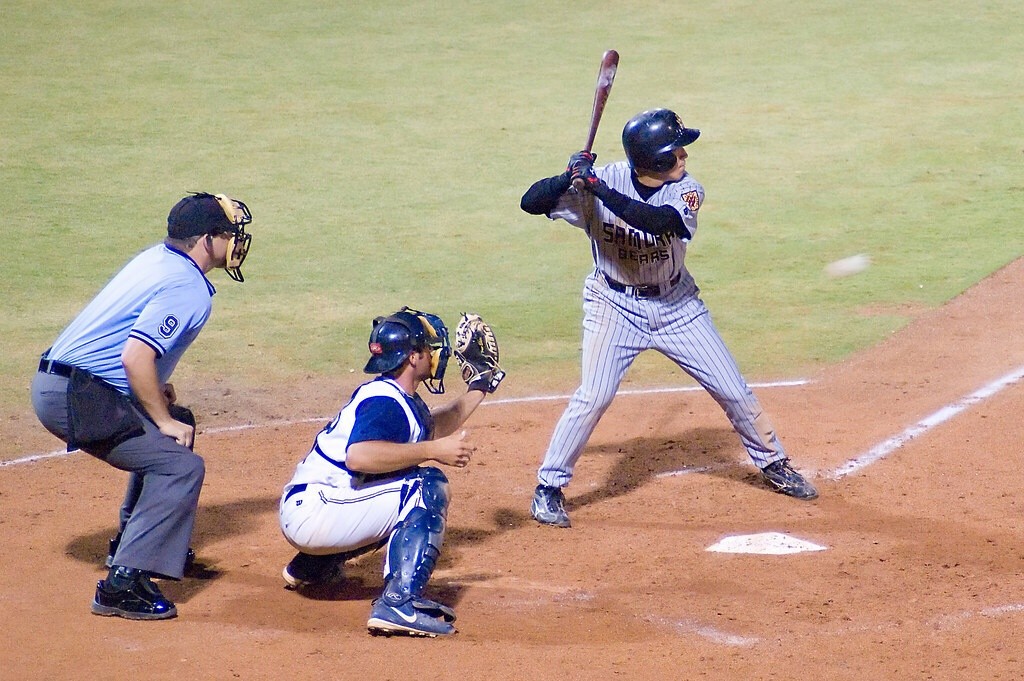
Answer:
<box><xmin>277</xmin><ymin>307</ymin><xmax>500</xmax><ymax>638</ymax></box>
<box><xmin>521</xmin><ymin>107</ymin><xmax>819</xmax><ymax>529</ymax></box>
<box><xmin>28</xmin><ymin>191</ymin><xmax>247</xmax><ymax>620</ymax></box>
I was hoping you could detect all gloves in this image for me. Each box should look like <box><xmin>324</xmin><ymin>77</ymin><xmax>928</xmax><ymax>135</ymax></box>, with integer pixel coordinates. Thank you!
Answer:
<box><xmin>571</xmin><ymin>165</ymin><xmax>609</xmax><ymax>199</ymax></box>
<box><xmin>566</xmin><ymin>150</ymin><xmax>597</xmax><ymax>175</ymax></box>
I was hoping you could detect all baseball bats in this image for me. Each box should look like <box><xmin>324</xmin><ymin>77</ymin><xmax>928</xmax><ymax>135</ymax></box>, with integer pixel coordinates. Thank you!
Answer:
<box><xmin>571</xmin><ymin>51</ymin><xmax>620</xmax><ymax>190</ymax></box>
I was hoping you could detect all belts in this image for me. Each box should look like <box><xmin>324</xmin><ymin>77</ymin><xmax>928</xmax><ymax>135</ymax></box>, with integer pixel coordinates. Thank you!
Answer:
<box><xmin>605</xmin><ymin>273</ymin><xmax>680</xmax><ymax>297</ymax></box>
<box><xmin>284</xmin><ymin>482</ymin><xmax>308</xmax><ymax>502</ymax></box>
<box><xmin>39</xmin><ymin>359</ymin><xmax>72</xmax><ymax>378</ymax></box>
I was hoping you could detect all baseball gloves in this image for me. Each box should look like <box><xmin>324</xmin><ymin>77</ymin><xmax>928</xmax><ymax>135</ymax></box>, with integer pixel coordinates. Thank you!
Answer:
<box><xmin>453</xmin><ymin>311</ymin><xmax>507</xmax><ymax>394</ymax></box>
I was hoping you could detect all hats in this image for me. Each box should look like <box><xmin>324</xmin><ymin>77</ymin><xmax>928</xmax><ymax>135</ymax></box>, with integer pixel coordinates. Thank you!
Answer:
<box><xmin>167</xmin><ymin>195</ymin><xmax>235</xmax><ymax>238</ymax></box>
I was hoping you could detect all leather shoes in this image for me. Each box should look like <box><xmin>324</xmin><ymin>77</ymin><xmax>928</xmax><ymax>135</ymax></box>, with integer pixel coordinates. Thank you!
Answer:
<box><xmin>89</xmin><ymin>580</ymin><xmax>177</xmax><ymax>619</ymax></box>
<box><xmin>106</xmin><ymin>539</ymin><xmax>194</xmax><ymax>568</ymax></box>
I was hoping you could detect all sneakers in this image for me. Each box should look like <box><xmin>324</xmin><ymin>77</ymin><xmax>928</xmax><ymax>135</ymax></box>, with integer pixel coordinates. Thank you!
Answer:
<box><xmin>763</xmin><ymin>457</ymin><xmax>819</xmax><ymax>500</ymax></box>
<box><xmin>282</xmin><ymin>556</ymin><xmax>340</xmax><ymax>587</ymax></box>
<box><xmin>531</xmin><ymin>484</ymin><xmax>571</xmax><ymax>527</ymax></box>
<box><xmin>366</xmin><ymin>600</ymin><xmax>457</xmax><ymax>637</ymax></box>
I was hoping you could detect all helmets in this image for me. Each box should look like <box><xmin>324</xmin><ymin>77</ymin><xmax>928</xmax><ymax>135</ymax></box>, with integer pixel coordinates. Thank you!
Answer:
<box><xmin>362</xmin><ymin>313</ymin><xmax>426</xmax><ymax>374</ymax></box>
<box><xmin>621</xmin><ymin>108</ymin><xmax>701</xmax><ymax>171</ymax></box>
